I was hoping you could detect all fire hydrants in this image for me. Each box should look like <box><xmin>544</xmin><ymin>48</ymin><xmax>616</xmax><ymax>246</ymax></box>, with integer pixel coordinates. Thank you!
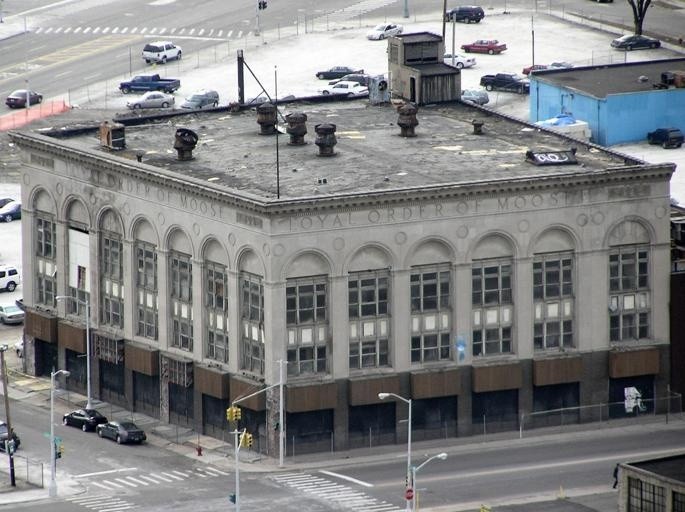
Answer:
<box><xmin>196</xmin><ymin>446</ymin><xmax>202</xmax><ymax>456</ymax></box>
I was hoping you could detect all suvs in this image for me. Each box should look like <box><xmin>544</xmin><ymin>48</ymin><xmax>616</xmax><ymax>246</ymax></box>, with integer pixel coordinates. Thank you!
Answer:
<box><xmin>647</xmin><ymin>127</ymin><xmax>685</xmax><ymax>148</ymax></box>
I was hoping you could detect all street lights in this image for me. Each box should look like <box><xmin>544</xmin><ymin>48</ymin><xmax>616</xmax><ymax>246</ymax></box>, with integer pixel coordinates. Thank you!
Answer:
<box><xmin>378</xmin><ymin>392</ymin><xmax>447</xmax><ymax>512</ymax></box>
<box><xmin>56</xmin><ymin>296</ymin><xmax>93</xmax><ymax>409</ymax></box>
<box><xmin>49</xmin><ymin>370</ymin><xmax>70</xmax><ymax>494</ymax></box>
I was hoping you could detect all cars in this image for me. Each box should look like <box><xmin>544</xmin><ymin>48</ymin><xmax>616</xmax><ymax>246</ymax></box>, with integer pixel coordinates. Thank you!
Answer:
<box><xmin>460</xmin><ymin>39</ymin><xmax>508</xmax><ymax>55</ymax></box>
<box><xmin>6</xmin><ymin>90</ymin><xmax>43</xmax><ymax>108</ymax></box>
<box><xmin>612</xmin><ymin>36</ymin><xmax>660</xmax><ymax>51</ymax></box>
<box><xmin>365</xmin><ymin>23</ymin><xmax>402</xmax><ymax>41</ymax></box>
<box><xmin>0</xmin><ymin>303</ymin><xmax>25</xmax><ymax>325</ymax></box>
<box><xmin>0</xmin><ymin>420</ymin><xmax>20</xmax><ymax>453</ymax></box>
<box><xmin>63</xmin><ymin>409</ymin><xmax>108</xmax><ymax>431</ymax></box>
<box><xmin>443</xmin><ymin>51</ymin><xmax>476</xmax><ymax>70</ymax></box>
<box><xmin>460</xmin><ymin>61</ymin><xmax>572</xmax><ymax>105</ymax></box>
<box><xmin>121</xmin><ymin>41</ymin><xmax>219</xmax><ymax>111</ymax></box>
<box><xmin>1</xmin><ymin>198</ymin><xmax>22</xmax><ymax>222</ymax></box>
<box><xmin>444</xmin><ymin>6</ymin><xmax>484</xmax><ymax>23</ymax></box>
<box><xmin>96</xmin><ymin>421</ymin><xmax>146</xmax><ymax>444</ymax></box>
<box><xmin>15</xmin><ymin>341</ymin><xmax>24</xmax><ymax>357</ymax></box>
<box><xmin>317</xmin><ymin>65</ymin><xmax>372</xmax><ymax>96</ymax></box>
<box><xmin>0</xmin><ymin>265</ymin><xmax>20</xmax><ymax>292</ymax></box>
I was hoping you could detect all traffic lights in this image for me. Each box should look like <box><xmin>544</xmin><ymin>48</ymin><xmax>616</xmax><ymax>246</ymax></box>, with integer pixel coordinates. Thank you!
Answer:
<box><xmin>60</xmin><ymin>440</ymin><xmax>64</xmax><ymax>454</ymax></box>
<box><xmin>262</xmin><ymin>1</ymin><xmax>267</xmax><ymax>9</ymax></box>
<box><xmin>259</xmin><ymin>1</ymin><xmax>262</xmax><ymax>9</ymax></box>
<box><xmin>238</xmin><ymin>435</ymin><xmax>252</xmax><ymax>450</ymax></box>
<box><xmin>226</xmin><ymin>408</ymin><xmax>241</xmax><ymax>422</ymax></box>
